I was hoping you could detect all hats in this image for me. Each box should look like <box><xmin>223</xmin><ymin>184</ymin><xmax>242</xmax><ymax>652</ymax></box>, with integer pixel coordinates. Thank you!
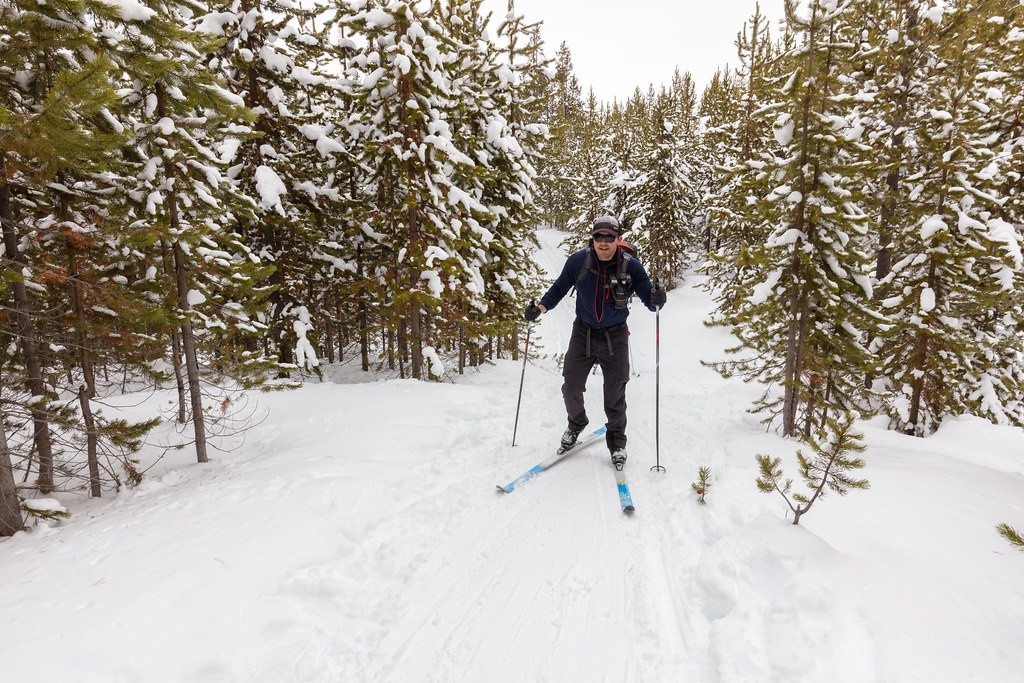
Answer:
<box><xmin>592</xmin><ymin>216</ymin><xmax>619</xmax><ymax>236</ymax></box>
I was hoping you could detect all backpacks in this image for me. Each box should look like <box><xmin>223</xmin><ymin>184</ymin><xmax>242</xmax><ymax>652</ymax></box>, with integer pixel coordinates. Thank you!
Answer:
<box><xmin>576</xmin><ymin>241</ymin><xmax>637</xmax><ymax>308</ymax></box>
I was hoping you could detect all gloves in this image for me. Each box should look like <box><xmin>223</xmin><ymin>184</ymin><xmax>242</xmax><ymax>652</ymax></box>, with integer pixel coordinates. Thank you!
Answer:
<box><xmin>525</xmin><ymin>305</ymin><xmax>541</xmax><ymax>320</ymax></box>
<box><xmin>651</xmin><ymin>276</ymin><xmax>666</xmax><ymax>304</ymax></box>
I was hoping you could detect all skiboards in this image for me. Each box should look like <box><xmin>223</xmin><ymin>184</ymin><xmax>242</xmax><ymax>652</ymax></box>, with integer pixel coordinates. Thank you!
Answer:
<box><xmin>495</xmin><ymin>424</ymin><xmax>636</xmax><ymax>514</ymax></box>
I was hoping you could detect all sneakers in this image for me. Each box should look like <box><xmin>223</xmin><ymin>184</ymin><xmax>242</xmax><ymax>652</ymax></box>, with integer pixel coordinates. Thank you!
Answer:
<box><xmin>561</xmin><ymin>419</ymin><xmax>589</xmax><ymax>449</ymax></box>
<box><xmin>609</xmin><ymin>448</ymin><xmax>627</xmax><ymax>464</ymax></box>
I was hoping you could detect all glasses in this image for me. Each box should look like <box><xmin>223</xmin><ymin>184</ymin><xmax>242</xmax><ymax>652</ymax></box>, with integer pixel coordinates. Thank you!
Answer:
<box><xmin>593</xmin><ymin>232</ymin><xmax>616</xmax><ymax>242</ymax></box>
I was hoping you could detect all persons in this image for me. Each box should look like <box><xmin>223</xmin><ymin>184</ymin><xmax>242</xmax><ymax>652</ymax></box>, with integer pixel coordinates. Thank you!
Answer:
<box><xmin>525</xmin><ymin>216</ymin><xmax>668</xmax><ymax>468</ymax></box>
